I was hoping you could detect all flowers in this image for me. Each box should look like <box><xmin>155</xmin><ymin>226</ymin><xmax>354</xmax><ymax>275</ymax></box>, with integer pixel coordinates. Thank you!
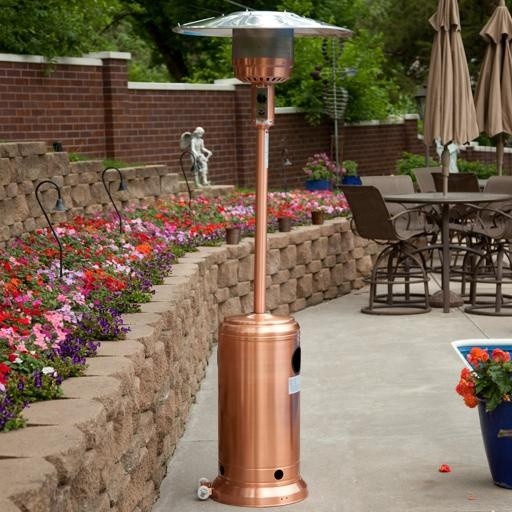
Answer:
<box><xmin>302</xmin><ymin>153</ymin><xmax>346</xmax><ymax>181</ymax></box>
<box><xmin>456</xmin><ymin>347</ymin><xmax>512</xmax><ymax>413</ymax></box>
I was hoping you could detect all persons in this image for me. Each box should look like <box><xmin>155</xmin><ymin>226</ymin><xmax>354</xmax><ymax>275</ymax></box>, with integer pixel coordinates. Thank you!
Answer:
<box><xmin>190</xmin><ymin>126</ymin><xmax>213</xmax><ymax>186</ymax></box>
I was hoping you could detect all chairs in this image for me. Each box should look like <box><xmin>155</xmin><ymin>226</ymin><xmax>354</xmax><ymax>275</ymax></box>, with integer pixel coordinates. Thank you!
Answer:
<box><xmin>335</xmin><ymin>166</ymin><xmax>512</xmax><ymax>317</ymax></box>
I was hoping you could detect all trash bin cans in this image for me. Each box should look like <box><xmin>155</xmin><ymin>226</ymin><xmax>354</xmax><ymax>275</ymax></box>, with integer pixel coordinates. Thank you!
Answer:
<box><xmin>209</xmin><ymin>312</ymin><xmax>309</xmax><ymax>508</ymax></box>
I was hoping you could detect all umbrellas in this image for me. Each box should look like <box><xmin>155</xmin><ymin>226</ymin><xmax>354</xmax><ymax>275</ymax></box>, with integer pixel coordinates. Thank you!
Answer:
<box><xmin>473</xmin><ymin>0</ymin><xmax>511</xmax><ymax>312</ymax></box>
<box><xmin>423</xmin><ymin>0</ymin><xmax>479</xmax><ymax>314</ymax></box>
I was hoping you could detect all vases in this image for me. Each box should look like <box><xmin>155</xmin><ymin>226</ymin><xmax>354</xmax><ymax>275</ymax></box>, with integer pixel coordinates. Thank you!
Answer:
<box><xmin>225</xmin><ymin>227</ymin><xmax>240</xmax><ymax>244</ymax></box>
<box><xmin>278</xmin><ymin>217</ymin><xmax>291</xmax><ymax>232</ymax></box>
<box><xmin>305</xmin><ymin>180</ymin><xmax>334</xmax><ymax>190</ymax></box>
<box><xmin>312</xmin><ymin>211</ymin><xmax>323</xmax><ymax>224</ymax></box>
<box><xmin>478</xmin><ymin>399</ymin><xmax>511</xmax><ymax>489</ymax></box>
